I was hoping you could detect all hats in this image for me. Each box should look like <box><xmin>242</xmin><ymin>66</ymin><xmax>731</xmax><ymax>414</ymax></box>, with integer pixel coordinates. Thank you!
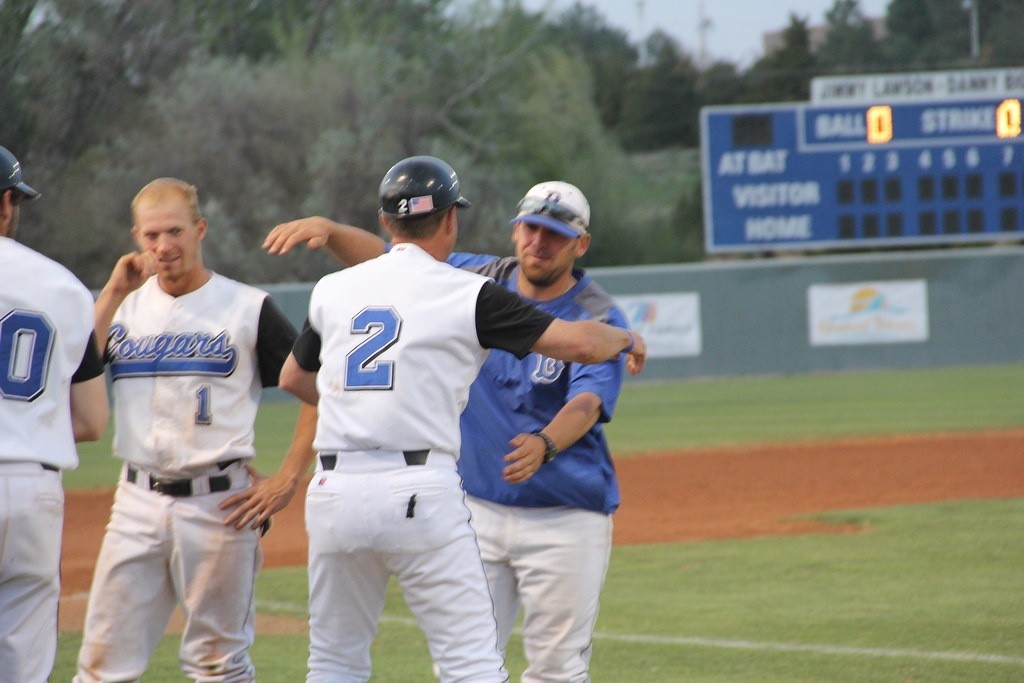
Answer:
<box><xmin>509</xmin><ymin>181</ymin><xmax>590</xmax><ymax>238</ymax></box>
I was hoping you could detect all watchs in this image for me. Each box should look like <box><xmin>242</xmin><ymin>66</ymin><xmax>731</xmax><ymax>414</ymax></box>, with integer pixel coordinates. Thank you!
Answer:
<box><xmin>533</xmin><ymin>433</ymin><xmax>558</xmax><ymax>465</ymax></box>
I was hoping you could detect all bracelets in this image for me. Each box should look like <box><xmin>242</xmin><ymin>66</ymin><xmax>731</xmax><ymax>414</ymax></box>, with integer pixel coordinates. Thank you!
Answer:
<box><xmin>620</xmin><ymin>329</ymin><xmax>634</xmax><ymax>353</ymax></box>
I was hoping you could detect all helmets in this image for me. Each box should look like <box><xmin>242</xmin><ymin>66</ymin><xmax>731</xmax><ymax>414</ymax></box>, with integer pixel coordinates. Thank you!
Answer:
<box><xmin>379</xmin><ymin>155</ymin><xmax>472</xmax><ymax>218</ymax></box>
<box><xmin>0</xmin><ymin>145</ymin><xmax>41</xmax><ymax>200</ymax></box>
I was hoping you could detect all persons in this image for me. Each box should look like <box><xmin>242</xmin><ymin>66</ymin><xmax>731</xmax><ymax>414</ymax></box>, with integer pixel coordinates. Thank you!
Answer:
<box><xmin>69</xmin><ymin>178</ymin><xmax>318</xmax><ymax>683</ymax></box>
<box><xmin>262</xmin><ymin>182</ymin><xmax>631</xmax><ymax>683</ymax></box>
<box><xmin>280</xmin><ymin>155</ymin><xmax>646</xmax><ymax>683</ymax></box>
<box><xmin>0</xmin><ymin>145</ymin><xmax>109</xmax><ymax>683</ymax></box>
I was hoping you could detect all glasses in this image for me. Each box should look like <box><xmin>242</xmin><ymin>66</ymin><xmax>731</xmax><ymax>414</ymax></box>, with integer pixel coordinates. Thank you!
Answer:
<box><xmin>517</xmin><ymin>196</ymin><xmax>588</xmax><ymax>227</ymax></box>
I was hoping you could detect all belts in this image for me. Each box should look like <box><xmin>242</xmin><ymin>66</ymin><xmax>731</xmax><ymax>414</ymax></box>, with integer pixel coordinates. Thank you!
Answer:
<box><xmin>128</xmin><ymin>467</ymin><xmax>230</xmax><ymax>496</ymax></box>
<box><xmin>42</xmin><ymin>463</ymin><xmax>60</xmax><ymax>472</ymax></box>
<box><xmin>320</xmin><ymin>449</ymin><xmax>431</xmax><ymax>470</ymax></box>
